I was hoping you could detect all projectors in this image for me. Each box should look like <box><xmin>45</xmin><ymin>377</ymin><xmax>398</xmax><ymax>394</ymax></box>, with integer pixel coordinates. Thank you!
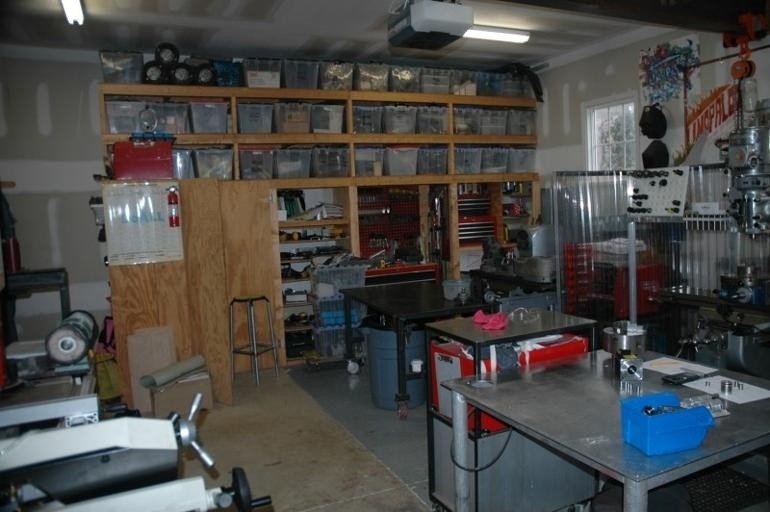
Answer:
<box><xmin>384</xmin><ymin>0</ymin><xmax>474</xmax><ymax>51</ymax></box>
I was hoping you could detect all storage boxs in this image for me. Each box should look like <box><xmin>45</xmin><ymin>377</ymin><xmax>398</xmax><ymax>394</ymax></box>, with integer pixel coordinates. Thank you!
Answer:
<box><xmin>311</xmin><ymin>258</ymin><xmax>370</xmax><ymax>361</ymax></box>
<box><xmin>152</xmin><ymin>371</ymin><xmax>212</xmax><ymax>420</ymax></box>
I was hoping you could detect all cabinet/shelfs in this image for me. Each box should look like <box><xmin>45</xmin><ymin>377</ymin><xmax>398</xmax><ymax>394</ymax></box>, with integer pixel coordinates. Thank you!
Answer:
<box><xmin>267</xmin><ymin>178</ymin><xmax>363</xmax><ymax>370</ymax></box>
<box><xmin>449</xmin><ymin>180</ymin><xmax>541</xmax><ymax>278</ymax></box>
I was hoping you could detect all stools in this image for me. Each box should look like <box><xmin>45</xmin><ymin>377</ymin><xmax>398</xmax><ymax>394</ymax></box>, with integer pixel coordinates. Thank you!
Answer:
<box><xmin>228</xmin><ymin>295</ymin><xmax>279</xmax><ymax>384</ymax></box>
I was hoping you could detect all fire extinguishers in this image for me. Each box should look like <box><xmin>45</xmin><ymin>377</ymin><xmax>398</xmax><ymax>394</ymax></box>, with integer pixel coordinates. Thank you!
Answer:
<box><xmin>166</xmin><ymin>186</ymin><xmax>180</xmax><ymax>228</ymax></box>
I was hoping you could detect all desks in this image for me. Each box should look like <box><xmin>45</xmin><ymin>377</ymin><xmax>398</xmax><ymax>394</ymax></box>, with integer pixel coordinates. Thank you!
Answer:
<box><xmin>440</xmin><ymin>348</ymin><xmax>768</xmax><ymax>511</ymax></box>
<box><xmin>341</xmin><ymin>278</ymin><xmax>564</xmax><ymax>419</ymax></box>
